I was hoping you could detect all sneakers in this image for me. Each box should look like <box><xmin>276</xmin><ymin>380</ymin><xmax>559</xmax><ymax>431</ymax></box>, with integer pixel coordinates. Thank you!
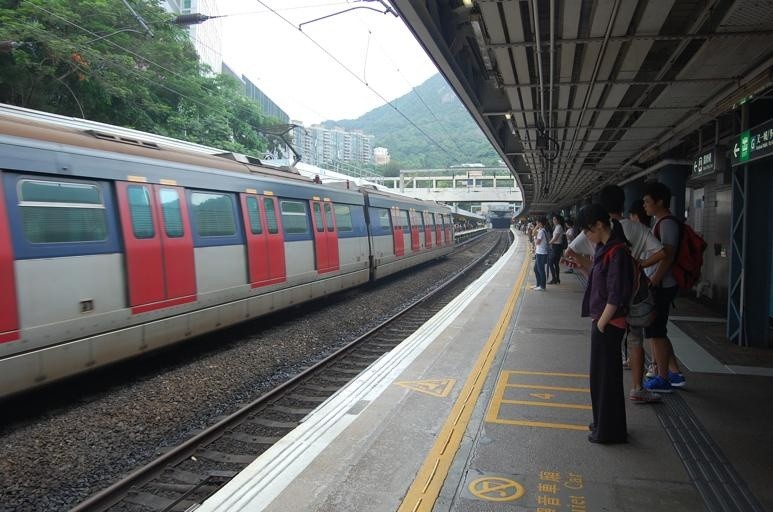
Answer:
<box><xmin>588</xmin><ymin>422</ymin><xmax>628</xmax><ymax>443</ymax></box>
<box><xmin>530</xmin><ymin>280</ymin><xmax>561</xmax><ymax>291</ymax></box>
<box><xmin>623</xmin><ymin>359</ymin><xmax>687</xmax><ymax>404</ymax></box>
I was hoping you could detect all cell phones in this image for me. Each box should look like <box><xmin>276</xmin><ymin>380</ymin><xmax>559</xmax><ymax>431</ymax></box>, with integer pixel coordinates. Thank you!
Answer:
<box><xmin>560</xmin><ymin>258</ymin><xmax>576</xmax><ymax>268</ymax></box>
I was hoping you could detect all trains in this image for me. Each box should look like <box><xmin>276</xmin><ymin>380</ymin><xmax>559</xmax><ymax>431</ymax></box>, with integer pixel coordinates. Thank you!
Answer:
<box><xmin>1</xmin><ymin>103</ymin><xmax>458</xmax><ymax>397</ymax></box>
<box><xmin>443</xmin><ymin>204</ymin><xmax>487</xmax><ymax>245</ymax></box>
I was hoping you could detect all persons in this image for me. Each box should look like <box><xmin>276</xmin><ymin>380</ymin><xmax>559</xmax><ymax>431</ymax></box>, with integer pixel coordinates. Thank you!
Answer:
<box><xmin>560</xmin><ymin>202</ymin><xmax>633</xmax><ymax>446</ymax></box>
<box><xmin>511</xmin><ymin>211</ymin><xmax>587</xmax><ymax>292</ymax></box>
<box><xmin>561</xmin><ymin>184</ymin><xmax>665</xmax><ymax>404</ymax></box>
<box><xmin>621</xmin><ymin>197</ymin><xmax>670</xmax><ymax>379</ymax></box>
<box><xmin>635</xmin><ymin>181</ymin><xmax>687</xmax><ymax>394</ymax></box>
<box><xmin>452</xmin><ymin>219</ymin><xmax>478</xmax><ymax>242</ymax></box>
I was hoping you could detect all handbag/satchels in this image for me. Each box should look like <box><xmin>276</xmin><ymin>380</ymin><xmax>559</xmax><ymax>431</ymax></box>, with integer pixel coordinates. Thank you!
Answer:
<box><xmin>562</xmin><ymin>234</ymin><xmax>568</xmax><ymax>249</ymax></box>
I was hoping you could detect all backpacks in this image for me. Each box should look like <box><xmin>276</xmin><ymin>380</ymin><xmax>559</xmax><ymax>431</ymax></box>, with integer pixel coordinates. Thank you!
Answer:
<box><xmin>603</xmin><ymin>245</ymin><xmax>657</xmax><ymax>327</ymax></box>
<box><xmin>653</xmin><ymin>215</ymin><xmax>707</xmax><ymax>291</ymax></box>
